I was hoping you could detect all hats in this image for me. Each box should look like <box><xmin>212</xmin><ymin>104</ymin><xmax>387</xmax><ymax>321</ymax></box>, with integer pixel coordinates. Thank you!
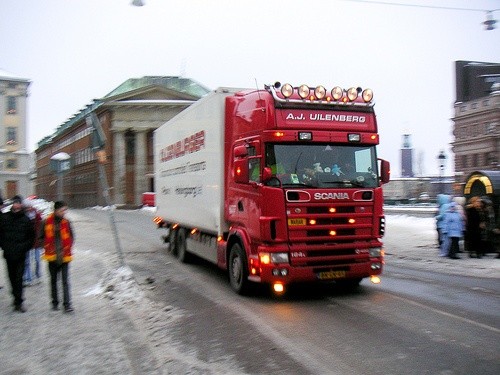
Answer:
<box><xmin>12</xmin><ymin>195</ymin><xmax>21</xmax><ymax>203</ymax></box>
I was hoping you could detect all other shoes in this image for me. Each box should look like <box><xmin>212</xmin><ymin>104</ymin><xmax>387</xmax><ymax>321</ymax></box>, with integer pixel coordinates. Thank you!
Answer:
<box><xmin>18</xmin><ymin>306</ymin><xmax>26</xmax><ymax>313</ymax></box>
<box><xmin>455</xmin><ymin>249</ymin><xmax>464</xmax><ymax>253</ymax></box>
<box><xmin>449</xmin><ymin>252</ymin><xmax>459</xmax><ymax>259</ymax></box>
<box><xmin>14</xmin><ymin>305</ymin><xmax>19</xmax><ymax>310</ymax></box>
<box><xmin>65</xmin><ymin>306</ymin><xmax>74</xmax><ymax>312</ymax></box>
<box><xmin>53</xmin><ymin>304</ymin><xmax>59</xmax><ymax>311</ymax></box>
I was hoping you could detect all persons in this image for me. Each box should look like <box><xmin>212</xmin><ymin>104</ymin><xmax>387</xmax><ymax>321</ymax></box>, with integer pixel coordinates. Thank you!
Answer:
<box><xmin>0</xmin><ymin>195</ymin><xmax>43</xmax><ymax>290</ymax></box>
<box><xmin>38</xmin><ymin>200</ymin><xmax>75</xmax><ymax>312</ymax></box>
<box><xmin>0</xmin><ymin>195</ymin><xmax>37</xmax><ymax>313</ymax></box>
<box><xmin>433</xmin><ymin>191</ymin><xmax>500</xmax><ymax>260</ymax></box>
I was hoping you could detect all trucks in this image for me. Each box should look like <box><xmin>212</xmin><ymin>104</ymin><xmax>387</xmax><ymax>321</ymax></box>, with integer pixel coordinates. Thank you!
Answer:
<box><xmin>153</xmin><ymin>81</ymin><xmax>392</xmax><ymax>296</ymax></box>
